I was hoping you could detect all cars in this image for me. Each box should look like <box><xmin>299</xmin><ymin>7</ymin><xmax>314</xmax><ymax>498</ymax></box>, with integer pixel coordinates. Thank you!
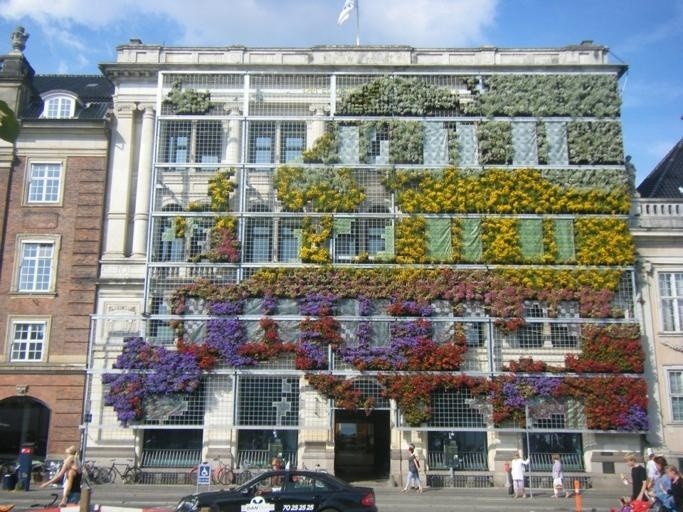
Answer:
<box><xmin>175</xmin><ymin>470</ymin><xmax>377</xmax><ymax>512</ymax></box>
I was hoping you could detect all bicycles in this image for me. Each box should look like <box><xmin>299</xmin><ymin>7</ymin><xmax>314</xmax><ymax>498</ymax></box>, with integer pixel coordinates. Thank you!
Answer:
<box><xmin>190</xmin><ymin>458</ymin><xmax>235</xmax><ymax>486</ymax></box>
<box><xmin>83</xmin><ymin>460</ymin><xmax>144</xmax><ymax>485</ymax></box>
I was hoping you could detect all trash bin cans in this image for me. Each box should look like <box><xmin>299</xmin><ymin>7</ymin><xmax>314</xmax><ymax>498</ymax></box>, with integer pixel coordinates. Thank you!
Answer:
<box><xmin>0</xmin><ymin>474</ymin><xmax>17</xmax><ymax>489</ymax></box>
<box><xmin>46</xmin><ymin>460</ymin><xmax>62</xmax><ymax>479</ymax></box>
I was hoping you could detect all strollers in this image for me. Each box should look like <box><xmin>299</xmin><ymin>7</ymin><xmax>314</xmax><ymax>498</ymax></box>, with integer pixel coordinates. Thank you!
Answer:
<box><xmin>611</xmin><ymin>496</ymin><xmax>657</xmax><ymax>512</ymax></box>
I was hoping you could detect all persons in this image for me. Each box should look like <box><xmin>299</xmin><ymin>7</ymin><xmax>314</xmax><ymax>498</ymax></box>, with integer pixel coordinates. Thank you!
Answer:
<box><xmin>271</xmin><ymin>452</ymin><xmax>283</xmax><ymax>486</ymax></box>
<box><xmin>625</xmin><ymin>155</ymin><xmax>637</xmax><ymax>194</ymax></box>
<box><xmin>337</xmin><ymin>0</ymin><xmax>356</xmax><ymax>26</ymax></box>
<box><xmin>83</xmin><ymin>461</ymin><xmax>94</xmax><ymax>485</ymax></box>
<box><xmin>552</xmin><ymin>455</ymin><xmax>570</xmax><ymax>499</ymax></box>
<box><xmin>622</xmin><ymin>448</ymin><xmax>683</xmax><ymax>512</ymax></box>
<box><xmin>507</xmin><ymin>451</ymin><xmax>529</xmax><ymax>498</ymax></box>
<box><xmin>402</xmin><ymin>444</ymin><xmax>428</xmax><ymax>494</ymax></box>
<box><xmin>257</xmin><ymin>474</ymin><xmax>281</xmax><ymax>492</ymax></box>
<box><xmin>40</xmin><ymin>447</ymin><xmax>82</xmax><ymax>509</ymax></box>
